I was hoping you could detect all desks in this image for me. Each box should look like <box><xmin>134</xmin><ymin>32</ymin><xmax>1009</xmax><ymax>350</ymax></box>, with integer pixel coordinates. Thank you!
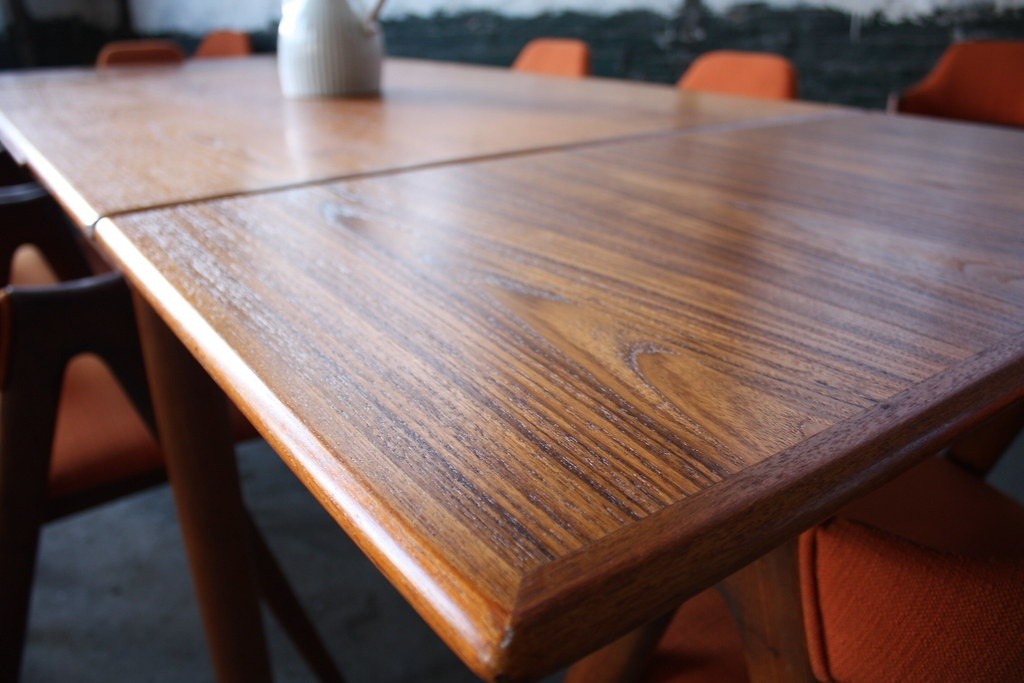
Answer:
<box><xmin>0</xmin><ymin>54</ymin><xmax>1024</xmax><ymax>683</ymax></box>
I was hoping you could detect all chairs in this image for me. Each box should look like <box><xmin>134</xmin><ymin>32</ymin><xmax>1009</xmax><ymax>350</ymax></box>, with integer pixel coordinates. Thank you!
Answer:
<box><xmin>195</xmin><ymin>32</ymin><xmax>250</xmax><ymax>57</ymax></box>
<box><xmin>677</xmin><ymin>50</ymin><xmax>799</xmax><ymax>103</ymax></box>
<box><xmin>93</xmin><ymin>40</ymin><xmax>185</xmax><ymax>68</ymax></box>
<box><xmin>551</xmin><ymin>447</ymin><xmax>1024</xmax><ymax>683</ymax></box>
<box><xmin>0</xmin><ymin>185</ymin><xmax>350</xmax><ymax>683</ymax></box>
<box><xmin>509</xmin><ymin>37</ymin><xmax>589</xmax><ymax>80</ymax></box>
<box><xmin>887</xmin><ymin>38</ymin><xmax>1023</xmax><ymax>131</ymax></box>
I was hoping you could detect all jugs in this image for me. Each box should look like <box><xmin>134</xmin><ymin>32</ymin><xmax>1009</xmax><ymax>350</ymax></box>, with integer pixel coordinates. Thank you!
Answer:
<box><xmin>278</xmin><ymin>0</ymin><xmax>391</xmax><ymax>100</ymax></box>
